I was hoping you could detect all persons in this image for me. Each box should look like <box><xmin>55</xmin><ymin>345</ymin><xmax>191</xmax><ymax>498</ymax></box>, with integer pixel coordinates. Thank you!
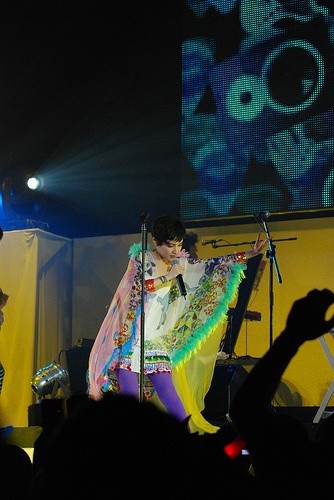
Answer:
<box><xmin>0</xmin><ymin>288</ymin><xmax>334</xmax><ymax>500</ymax></box>
<box><xmin>0</xmin><ymin>224</ymin><xmax>15</xmax><ymax>447</ymax></box>
<box><xmin>84</xmin><ymin>215</ymin><xmax>274</xmax><ymax>443</ymax></box>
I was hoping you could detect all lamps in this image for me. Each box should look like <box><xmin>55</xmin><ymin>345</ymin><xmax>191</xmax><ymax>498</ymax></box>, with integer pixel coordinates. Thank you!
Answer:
<box><xmin>29</xmin><ymin>361</ymin><xmax>70</xmax><ymax>404</ymax></box>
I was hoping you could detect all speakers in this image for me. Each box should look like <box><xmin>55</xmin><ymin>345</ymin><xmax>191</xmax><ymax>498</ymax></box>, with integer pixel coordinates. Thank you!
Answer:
<box><xmin>27</xmin><ymin>398</ymin><xmax>65</xmax><ymax>447</ymax></box>
<box><xmin>64</xmin><ymin>344</ymin><xmax>93</xmax><ymax>399</ymax></box>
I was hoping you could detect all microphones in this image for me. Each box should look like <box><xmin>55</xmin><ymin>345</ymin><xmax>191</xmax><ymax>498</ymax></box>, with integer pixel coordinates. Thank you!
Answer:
<box><xmin>201</xmin><ymin>238</ymin><xmax>223</xmax><ymax>245</ymax></box>
<box><xmin>170</xmin><ymin>257</ymin><xmax>187</xmax><ymax>296</ymax></box>
